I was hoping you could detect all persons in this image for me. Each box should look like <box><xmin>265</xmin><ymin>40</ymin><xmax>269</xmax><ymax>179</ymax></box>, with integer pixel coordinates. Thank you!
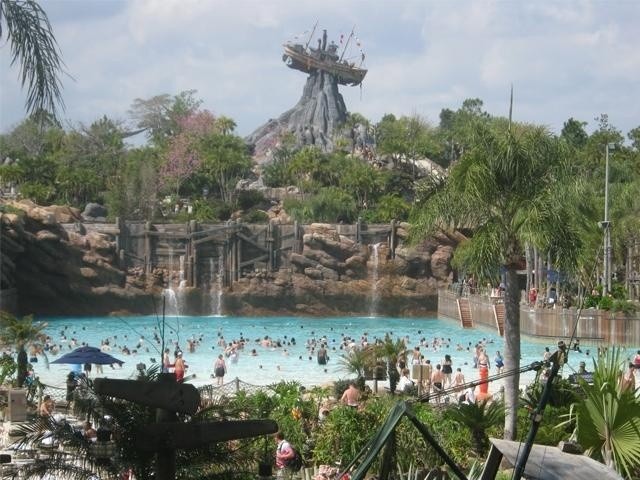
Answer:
<box><xmin>1</xmin><ymin>323</ymin><xmax>639</xmax><ymax>424</ymax></box>
<box><xmin>272</xmin><ymin>430</ymin><xmax>297</xmax><ymax>480</ymax></box>
<box><xmin>460</xmin><ymin>274</ymin><xmax>616</xmax><ymax>311</ymax></box>
<box><xmin>82</xmin><ymin>421</ymin><xmax>99</xmax><ymax>438</ymax></box>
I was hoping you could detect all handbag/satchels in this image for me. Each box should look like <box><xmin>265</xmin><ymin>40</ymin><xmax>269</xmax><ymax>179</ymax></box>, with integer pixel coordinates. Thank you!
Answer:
<box><xmin>283</xmin><ymin>448</ymin><xmax>302</xmax><ymax>472</ymax></box>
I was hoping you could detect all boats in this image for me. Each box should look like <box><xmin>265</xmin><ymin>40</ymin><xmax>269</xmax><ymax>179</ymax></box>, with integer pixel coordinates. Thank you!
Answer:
<box><xmin>282</xmin><ymin>44</ymin><xmax>368</xmax><ymax>87</ymax></box>
